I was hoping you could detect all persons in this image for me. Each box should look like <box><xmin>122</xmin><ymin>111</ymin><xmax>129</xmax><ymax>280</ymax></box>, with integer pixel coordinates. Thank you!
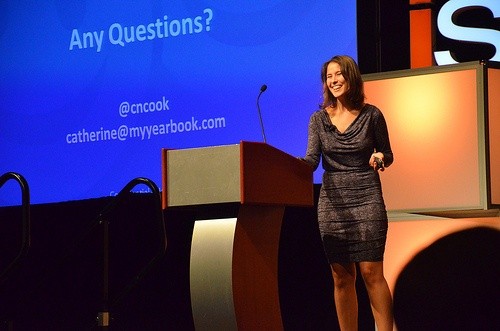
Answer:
<box><xmin>295</xmin><ymin>54</ymin><xmax>394</xmax><ymax>331</ymax></box>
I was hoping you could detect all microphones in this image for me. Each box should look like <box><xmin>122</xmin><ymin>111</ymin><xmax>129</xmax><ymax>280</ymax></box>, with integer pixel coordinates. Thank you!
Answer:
<box><xmin>256</xmin><ymin>84</ymin><xmax>268</xmax><ymax>143</ymax></box>
